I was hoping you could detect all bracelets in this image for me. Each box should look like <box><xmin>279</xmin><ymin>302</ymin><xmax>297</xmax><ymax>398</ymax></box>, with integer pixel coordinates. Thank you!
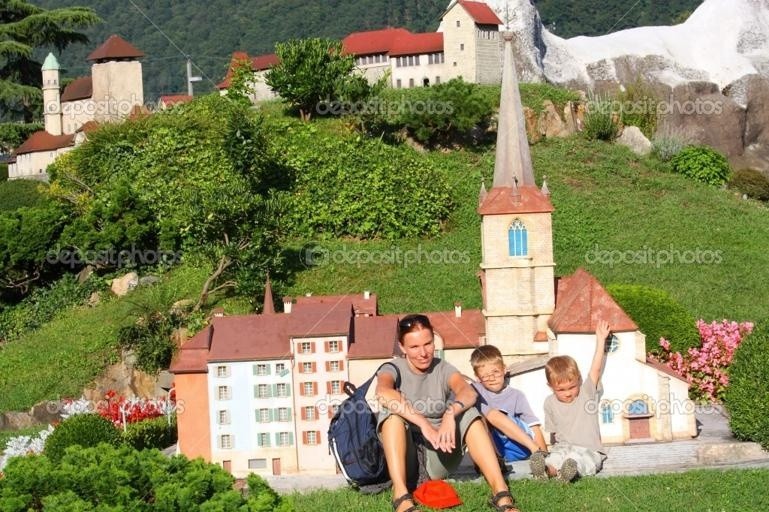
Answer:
<box><xmin>453</xmin><ymin>399</ymin><xmax>467</xmax><ymax>409</ymax></box>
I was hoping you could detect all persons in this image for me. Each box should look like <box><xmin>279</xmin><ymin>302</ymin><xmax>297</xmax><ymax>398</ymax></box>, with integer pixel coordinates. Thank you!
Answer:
<box><xmin>528</xmin><ymin>319</ymin><xmax>614</xmax><ymax>483</ymax></box>
<box><xmin>374</xmin><ymin>312</ymin><xmax>521</xmax><ymax>511</ymax></box>
<box><xmin>446</xmin><ymin>343</ymin><xmax>551</xmax><ymax>481</ymax></box>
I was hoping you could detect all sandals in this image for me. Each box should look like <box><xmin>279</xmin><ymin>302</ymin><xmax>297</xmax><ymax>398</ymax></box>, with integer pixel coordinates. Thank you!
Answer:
<box><xmin>488</xmin><ymin>492</ymin><xmax>520</xmax><ymax>512</ymax></box>
<box><xmin>390</xmin><ymin>493</ymin><xmax>419</xmax><ymax>511</ymax></box>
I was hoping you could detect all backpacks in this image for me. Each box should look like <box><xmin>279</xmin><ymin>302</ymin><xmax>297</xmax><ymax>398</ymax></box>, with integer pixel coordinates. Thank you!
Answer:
<box><xmin>328</xmin><ymin>362</ymin><xmax>401</xmax><ymax>495</ymax></box>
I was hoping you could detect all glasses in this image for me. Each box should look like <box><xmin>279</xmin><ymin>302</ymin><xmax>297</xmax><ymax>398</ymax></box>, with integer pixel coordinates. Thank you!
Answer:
<box><xmin>476</xmin><ymin>366</ymin><xmax>506</xmax><ymax>383</ymax></box>
<box><xmin>400</xmin><ymin>315</ymin><xmax>430</xmax><ymax>329</ymax></box>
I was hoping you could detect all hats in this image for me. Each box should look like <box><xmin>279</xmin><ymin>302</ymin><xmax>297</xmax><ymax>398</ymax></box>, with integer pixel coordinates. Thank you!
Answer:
<box><xmin>413</xmin><ymin>481</ymin><xmax>462</xmax><ymax>508</ymax></box>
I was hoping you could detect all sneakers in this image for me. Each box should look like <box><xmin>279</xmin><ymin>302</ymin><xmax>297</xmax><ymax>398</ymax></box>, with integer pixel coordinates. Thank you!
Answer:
<box><xmin>472</xmin><ymin>452</ymin><xmax>507</xmax><ymax>475</ymax></box>
<box><xmin>530</xmin><ymin>454</ymin><xmax>545</xmax><ymax>479</ymax></box>
<box><xmin>558</xmin><ymin>459</ymin><xmax>578</xmax><ymax>483</ymax></box>
